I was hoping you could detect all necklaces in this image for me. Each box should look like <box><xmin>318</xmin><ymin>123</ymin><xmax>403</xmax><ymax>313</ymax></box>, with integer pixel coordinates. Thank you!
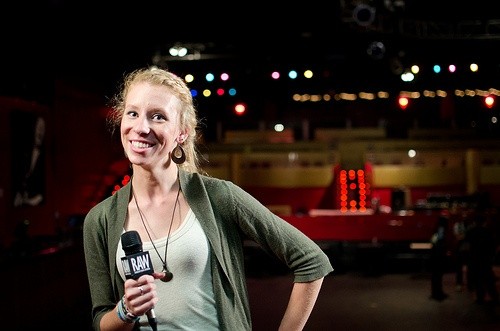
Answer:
<box><xmin>133</xmin><ymin>187</ymin><xmax>182</xmax><ymax>282</ymax></box>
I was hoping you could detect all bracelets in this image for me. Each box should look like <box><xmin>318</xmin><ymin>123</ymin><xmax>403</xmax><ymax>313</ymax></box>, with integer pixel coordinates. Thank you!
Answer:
<box><xmin>116</xmin><ymin>296</ymin><xmax>138</xmax><ymax>324</ymax></box>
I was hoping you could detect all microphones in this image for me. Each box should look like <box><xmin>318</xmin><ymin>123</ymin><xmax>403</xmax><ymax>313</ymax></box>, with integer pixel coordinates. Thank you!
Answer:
<box><xmin>120</xmin><ymin>231</ymin><xmax>158</xmax><ymax>331</ymax></box>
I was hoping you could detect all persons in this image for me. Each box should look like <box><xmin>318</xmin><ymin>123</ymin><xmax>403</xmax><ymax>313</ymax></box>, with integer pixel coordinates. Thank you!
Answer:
<box><xmin>83</xmin><ymin>66</ymin><xmax>335</xmax><ymax>331</ymax></box>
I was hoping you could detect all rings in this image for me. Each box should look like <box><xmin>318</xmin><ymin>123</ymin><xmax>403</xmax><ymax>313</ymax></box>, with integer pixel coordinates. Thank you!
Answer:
<box><xmin>139</xmin><ymin>287</ymin><xmax>143</xmax><ymax>294</ymax></box>
